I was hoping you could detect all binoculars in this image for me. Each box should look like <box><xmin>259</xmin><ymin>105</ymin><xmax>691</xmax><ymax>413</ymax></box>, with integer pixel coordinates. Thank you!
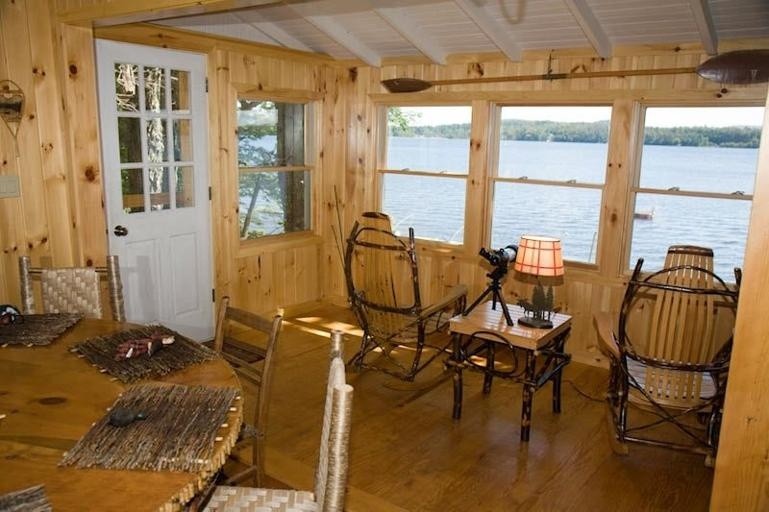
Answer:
<box><xmin>480</xmin><ymin>245</ymin><xmax>518</xmax><ymax>266</ymax></box>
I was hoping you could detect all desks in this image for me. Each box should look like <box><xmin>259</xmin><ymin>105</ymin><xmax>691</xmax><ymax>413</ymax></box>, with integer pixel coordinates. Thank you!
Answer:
<box><xmin>446</xmin><ymin>298</ymin><xmax>575</xmax><ymax>442</ymax></box>
<box><xmin>1</xmin><ymin>315</ymin><xmax>244</xmax><ymax>512</ymax></box>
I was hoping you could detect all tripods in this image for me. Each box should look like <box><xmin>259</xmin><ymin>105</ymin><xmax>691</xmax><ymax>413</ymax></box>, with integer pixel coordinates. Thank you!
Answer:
<box><xmin>462</xmin><ymin>272</ymin><xmax>516</xmax><ymax>328</ymax></box>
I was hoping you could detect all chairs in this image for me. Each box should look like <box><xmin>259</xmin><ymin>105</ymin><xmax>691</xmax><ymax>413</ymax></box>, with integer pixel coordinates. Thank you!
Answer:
<box><xmin>594</xmin><ymin>236</ymin><xmax>742</xmax><ymax>473</ymax></box>
<box><xmin>199</xmin><ymin>296</ymin><xmax>355</xmax><ymax>512</ymax></box>
<box><xmin>342</xmin><ymin>212</ymin><xmax>467</xmax><ymax>382</ymax></box>
<box><xmin>16</xmin><ymin>257</ymin><xmax>124</xmax><ymax>323</ymax></box>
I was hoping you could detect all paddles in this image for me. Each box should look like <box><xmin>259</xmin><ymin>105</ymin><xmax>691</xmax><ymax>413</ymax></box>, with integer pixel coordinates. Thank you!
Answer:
<box><xmin>379</xmin><ymin>49</ymin><xmax>769</xmax><ymax>93</ymax></box>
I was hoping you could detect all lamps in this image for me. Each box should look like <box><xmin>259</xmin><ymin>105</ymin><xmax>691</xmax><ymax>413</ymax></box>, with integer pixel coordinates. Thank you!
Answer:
<box><xmin>511</xmin><ymin>235</ymin><xmax>567</xmax><ymax>330</ymax></box>
